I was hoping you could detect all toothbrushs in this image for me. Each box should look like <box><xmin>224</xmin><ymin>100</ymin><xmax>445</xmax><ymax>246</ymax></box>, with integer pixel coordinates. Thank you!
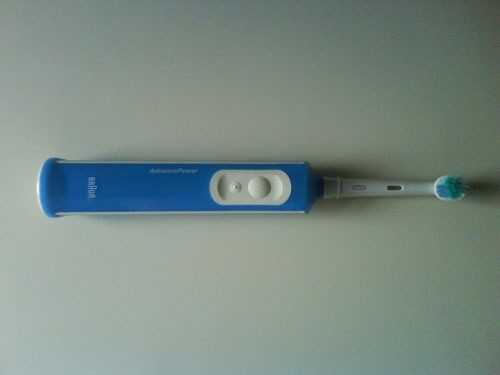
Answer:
<box><xmin>36</xmin><ymin>156</ymin><xmax>469</xmax><ymax>219</ymax></box>
